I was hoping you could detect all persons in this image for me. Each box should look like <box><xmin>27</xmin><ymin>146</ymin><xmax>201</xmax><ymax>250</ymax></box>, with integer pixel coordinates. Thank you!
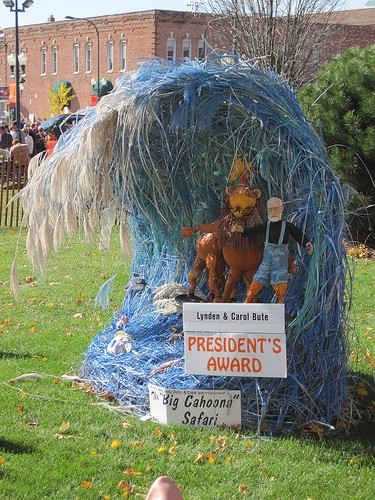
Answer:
<box><xmin>53</xmin><ymin>122</ymin><xmax>61</xmax><ymax>140</ymax></box>
<box><xmin>24</xmin><ymin>130</ymin><xmax>33</xmax><ymax>156</ymax></box>
<box><xmin>10</xmin><ymin>121</ymin><xmax>24</xmax><ymax>144</ymax></box>
<box><xmin>0</xmin><ymin>126</ymin><xmax>13</xmax><ymax>149</ymax></box>
<box><xmin>3</xmin><ymin>125</ymin><xmax>10</xmax><ymax>135</ymax></box>
<box><xmin>10</xmin><ymin>139</ymin><xmax>30</xmax><ymax>167</ymax></box>
<box><xmin>231</xmin><ymin>197</ymin><xmax>313</xmax><ymax>303</ymax></box>
<box><xmin>36</xmin><ymin>128</ymin><xmax>46</xmax><ymax>152</ymax></box>
<box><xmin>29</xmin><ymin>124</ymin><xmax>39</xmax><ymax>155</ymax></box>
<box><xmin>44</xmin><ymin>128</ymin><xmax>57</xmax><ymax>141</ymax></box>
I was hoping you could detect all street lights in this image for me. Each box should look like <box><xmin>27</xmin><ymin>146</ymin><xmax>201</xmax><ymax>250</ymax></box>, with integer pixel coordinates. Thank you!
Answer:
<box><xmin>65</xmin><ymin>15</ymin><xmax>101</xmax><ymax>101</ymax></box>
<box><xmin>202</xmin><ymin>14</ymin><xmax>236</xmax><ymax>64</ymax></box>
<box><xmin>3</xmin><ymin>0</ymin><xmax>34</xmax><ymax>129</ymax></box>
